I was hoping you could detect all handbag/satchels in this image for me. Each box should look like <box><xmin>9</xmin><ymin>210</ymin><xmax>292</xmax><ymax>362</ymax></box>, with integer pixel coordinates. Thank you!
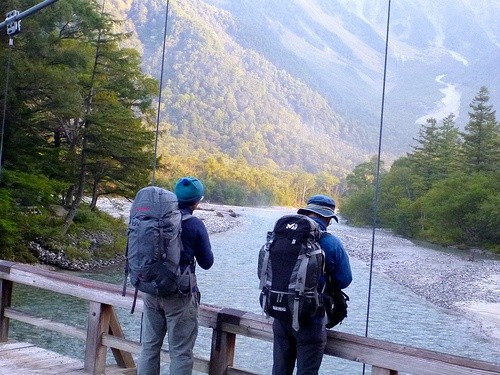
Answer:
<box><xmin>325</xmin><ymin>291</ymin><xmax>349</xmax><ymax>329</ymax></box>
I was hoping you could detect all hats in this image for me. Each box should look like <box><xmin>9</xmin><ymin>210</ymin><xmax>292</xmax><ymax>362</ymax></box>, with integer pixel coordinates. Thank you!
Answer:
<box><xmin>173</xmin><ymin>177</ymin><xmax>203</xmax><ymax>207</ymax></box>
<box><xmin>296</xmin><ymin>194</ymin><xmax>339</xmax><ymax>224</ymax></box>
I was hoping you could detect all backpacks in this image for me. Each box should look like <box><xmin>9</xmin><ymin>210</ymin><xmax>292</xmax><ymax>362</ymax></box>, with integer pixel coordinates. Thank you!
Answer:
<box><xmin>121</xmin><ymin>186</ymin><xmax>199</xmax><ymax>297</ymax></box>
<box><xmin>256</xmin><ymin>214</ymin><xmax>329</xmax><ymax>332</ymax></box>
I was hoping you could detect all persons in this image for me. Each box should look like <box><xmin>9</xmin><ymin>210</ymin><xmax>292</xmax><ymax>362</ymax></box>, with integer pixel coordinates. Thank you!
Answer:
<box><xmin>138</xmin><ymin>176</ymin><xmax>215</xmax><ymax>375</ymax></box>
<box><xmin>271</xmin><ymin>195</ymin><xmax>354</xmax><ymax>375</ymax></box>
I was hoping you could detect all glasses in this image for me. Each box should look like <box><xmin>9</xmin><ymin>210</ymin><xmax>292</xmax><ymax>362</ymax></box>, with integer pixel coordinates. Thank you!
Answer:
<box><xmin>200</xmin><ymin>196</ymin><xmax>204</xmax><ymax>201</ymax></box>
<box><xmin>329</xmin><ymin>220</ymin><xmax>332</xmax><ymax>225</ymax></box>
